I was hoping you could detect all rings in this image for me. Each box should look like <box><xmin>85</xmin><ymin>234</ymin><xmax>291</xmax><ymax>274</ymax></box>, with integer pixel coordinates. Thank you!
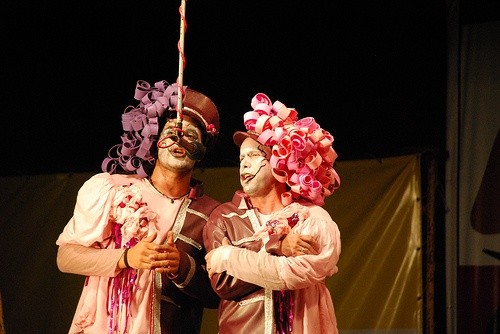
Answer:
<box><xmin>301</xmin><ymin>248</ymin><xmax>304</xmax><ymax>252</ymax></box>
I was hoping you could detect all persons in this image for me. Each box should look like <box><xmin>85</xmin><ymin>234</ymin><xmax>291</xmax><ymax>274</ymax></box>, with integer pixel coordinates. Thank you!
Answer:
<box><xmin>56</xmin><ymin>80</ymin><xmax>221</xmax><ymax>334</ymax></box>
<box><xmin>203</xmin><ymin>93</ymin><xmax>339</xmax><ymax>334</ymax></box>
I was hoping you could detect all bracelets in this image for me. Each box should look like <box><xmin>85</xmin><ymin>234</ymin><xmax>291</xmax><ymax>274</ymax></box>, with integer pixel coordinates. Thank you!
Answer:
<box><xmin>124</xmin><ymin>248</ymin><xmax>131</xmax><ymax>269</ymax></box>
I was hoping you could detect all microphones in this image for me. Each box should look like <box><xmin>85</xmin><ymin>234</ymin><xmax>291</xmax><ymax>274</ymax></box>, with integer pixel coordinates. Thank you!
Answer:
<box><xmin>171</xmin><ymin>134</ymin><xmax>205</xmax><ymax>160</ymax></box>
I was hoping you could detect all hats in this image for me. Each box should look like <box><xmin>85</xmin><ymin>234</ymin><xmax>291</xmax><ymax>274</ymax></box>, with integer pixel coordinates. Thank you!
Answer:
<box><xmin>230</xmin><ymin>91</ymin><xmax>342</xmax><ymax>206</ymax></box>
<box><xmin>100</xmin><ymin>78</ymin><xmax>222</xmax><ymax>174</ymax></box>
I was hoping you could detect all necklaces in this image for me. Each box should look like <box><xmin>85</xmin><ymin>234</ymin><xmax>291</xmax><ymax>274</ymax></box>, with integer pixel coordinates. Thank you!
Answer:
<box><xmin>148</xmin><ymin>176</ymin><xmax>189</xmax><ymax>204</ymax></box>
